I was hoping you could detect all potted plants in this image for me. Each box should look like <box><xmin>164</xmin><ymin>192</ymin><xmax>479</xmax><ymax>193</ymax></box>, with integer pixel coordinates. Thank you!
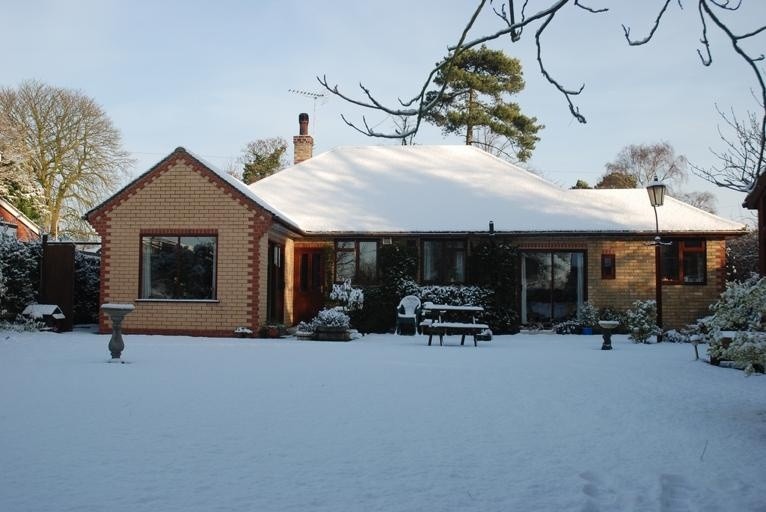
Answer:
<box><xmin>316</xmin><ymin>309</ymin><xmax>350</xmax><ymax>341</ymax></box>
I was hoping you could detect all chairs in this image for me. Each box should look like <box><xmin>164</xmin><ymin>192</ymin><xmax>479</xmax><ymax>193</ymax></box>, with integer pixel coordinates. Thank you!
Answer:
<box><xmin>395</xmin><ymin>295</ymin><xmax>421</xmax><ymax>335</ymax></box>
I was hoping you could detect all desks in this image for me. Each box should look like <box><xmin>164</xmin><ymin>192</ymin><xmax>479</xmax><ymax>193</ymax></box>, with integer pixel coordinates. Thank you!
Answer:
<box><xmin>425</xmin><ymin>305</ymin><xmax>484</xmax><ymax>345</ymax></box>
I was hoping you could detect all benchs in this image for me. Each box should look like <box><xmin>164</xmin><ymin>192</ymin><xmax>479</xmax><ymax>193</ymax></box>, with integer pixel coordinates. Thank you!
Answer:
<box><xmin>433</xmin><ymin>323</ymin><xmax>489</xmax><ymax>347</ymax></box>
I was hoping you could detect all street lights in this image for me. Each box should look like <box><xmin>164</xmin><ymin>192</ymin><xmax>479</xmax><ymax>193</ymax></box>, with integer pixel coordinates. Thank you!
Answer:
<box><xmin>644</xmin><ymin>171</ymin><xmax>665</xmax><ymax>344</ymax></box>
